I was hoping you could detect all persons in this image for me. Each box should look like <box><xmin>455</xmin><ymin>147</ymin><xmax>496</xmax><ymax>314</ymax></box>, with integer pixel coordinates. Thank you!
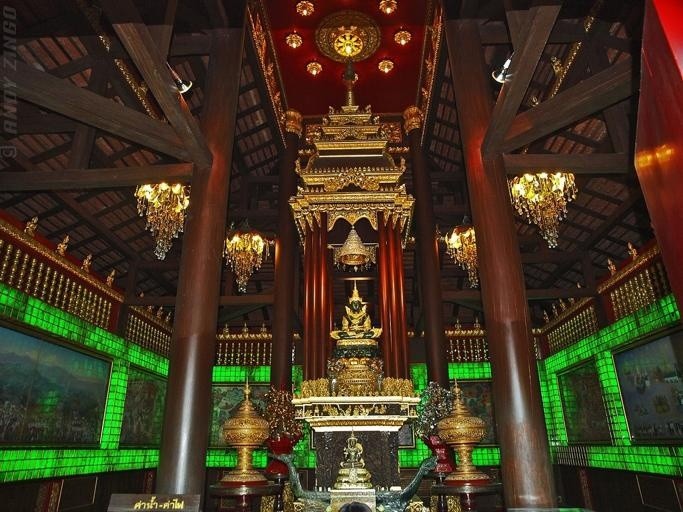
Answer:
<box><xmin>337</xmin><ymin>501</ymin><xmax>372</xmax><ymax>512</ymax></box>
<box><xmin>336</xmin><ymin>279</ymin><xmax>374</xmax><ymax>338</ymax></box>
<box><xmin>340</xmin><ymin>427</ymin><xmax>366</xmax><ymax>468</ymax></box>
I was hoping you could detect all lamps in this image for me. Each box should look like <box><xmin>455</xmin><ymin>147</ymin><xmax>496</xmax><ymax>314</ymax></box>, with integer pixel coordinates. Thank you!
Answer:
<box><xmin>166</xmin><ymin>62</ymin><xmax>193</xmax><ymax>95</ymax></box>
<box><xmin>506</xmin><ymin>169</ymin><xmax>578</xmax><ymax>249</ymax></box>
<box><xmin>444</xmin><ymin>224</ymin><xmax>481</xmax><ymax>289</ymax></box>
<box><xmin>286</xmin><ymin>0</ymin><xmax>412</xmax><ymax>76</ymax></box>
<box><xmin>490</xmin><ymin>49</ymin><xmax>516</xmax><ymax>83</ymax></box>
<box><xmin>221</xmin><ymin>224</ymin><xmax>270</xmax><ymax>294</ymax></box>
<box><xmin>134</xmin><ymin>181</ymin><xmax>190</xmax><ymax>262</ymax></box>
